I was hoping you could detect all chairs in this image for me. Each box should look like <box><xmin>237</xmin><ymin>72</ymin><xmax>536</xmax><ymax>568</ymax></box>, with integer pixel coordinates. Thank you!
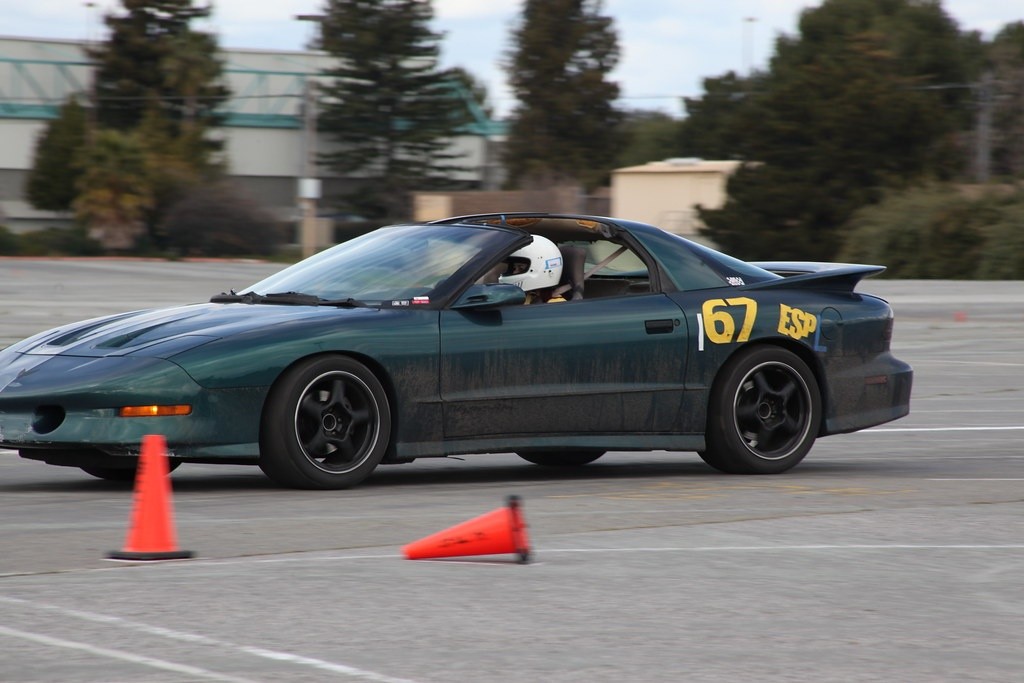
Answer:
<box><xmin>555</xmin><ymin>244</ymin><xmax>588</xmax><ymax>305</ymax></box>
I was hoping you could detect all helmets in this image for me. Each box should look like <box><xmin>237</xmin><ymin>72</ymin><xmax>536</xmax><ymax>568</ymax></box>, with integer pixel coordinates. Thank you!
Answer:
<box><xmin>498</xmin><ymin>234</ymin><xmax>564</xmax><ymax>292</ymax></box>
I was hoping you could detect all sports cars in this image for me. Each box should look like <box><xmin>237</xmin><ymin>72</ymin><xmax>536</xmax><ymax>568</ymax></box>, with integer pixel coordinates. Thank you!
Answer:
<box><xmin>1</xmin><ymin>211</ymin><xmax>915</xmax><ymax>492</ymax></box>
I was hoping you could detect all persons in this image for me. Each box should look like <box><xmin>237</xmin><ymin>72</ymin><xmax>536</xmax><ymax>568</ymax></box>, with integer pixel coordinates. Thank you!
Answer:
<box><xmin>498</xmin><ymin>234</ymin><xmax>566</xmax><ymax>305</ymax></box>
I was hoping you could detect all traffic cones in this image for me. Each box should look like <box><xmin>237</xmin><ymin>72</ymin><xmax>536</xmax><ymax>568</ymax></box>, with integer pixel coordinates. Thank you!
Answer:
<box><xmin>402</xmin><ymin>494</ymin><xmax>531</xmax><ymax>566</ymax></box>
<box><xmin>102</xmin><ymin>435</ymin><xmax>198</xmax><ymax>561</ymax></box>
